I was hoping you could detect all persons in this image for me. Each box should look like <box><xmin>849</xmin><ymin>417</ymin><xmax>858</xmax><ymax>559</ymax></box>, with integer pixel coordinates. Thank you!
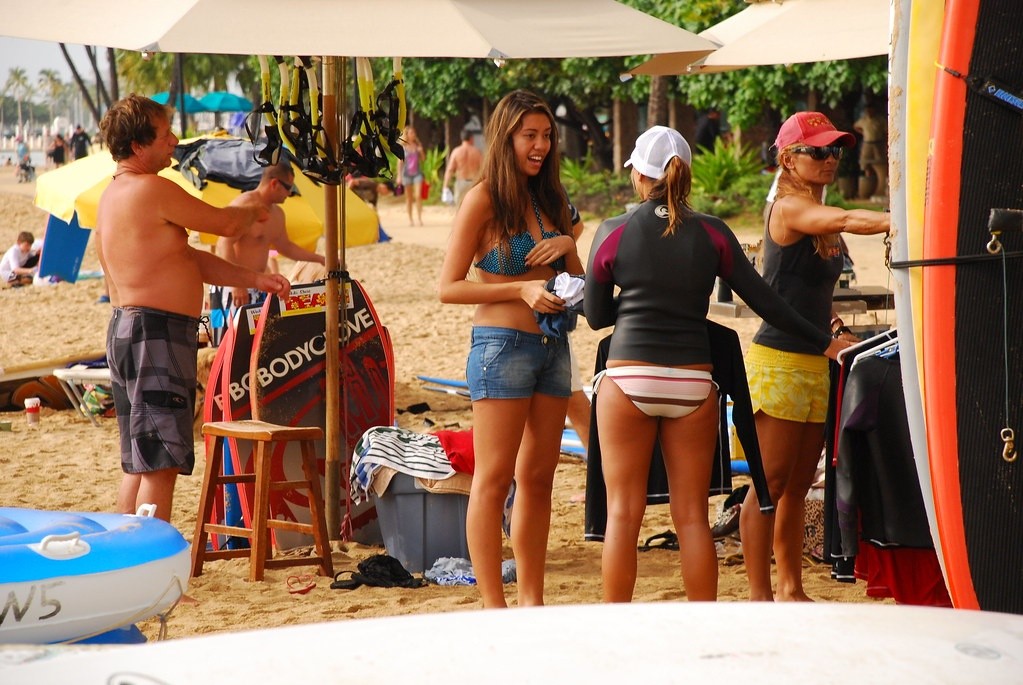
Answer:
<box><xmin>763</xmin><ymin>165</ymin><xmax>826</xmax><ymax>218</ymax></box>
<box><xmin>440</xmin><ymin>88</ymin><xmax>586</xmax><ymax>608</ymax></box>
<box><xmin>95</xmin><ymin>96</ymin><xmax>290</xmax><ymax>521</ymax></box>
<box><xmin>3</xmin><ymin>136</ymin><xmax>34</xmax><ymax>183</ymax></box>
<box><xmin>396</xmin><ymin>126</ymin><xmax>425</xmax><ymax>227</ymax></box>
<box><xmin>695</xmin><ymin>109</ymin><xmax>720</xmax><ymax>154</ymax></box>
<box><xmin>836</xmin><ymin>104</ymin><xmax>887</xmax><ymax>199</ymax></box>
<box><xmin>0</xmin><ymin>231</ymin><xmax>40</xmax><ymax>288</ymax></box>
<box><xmin>44</xmin><ymin>124</ymin><xmax>103</xmax><ymax>166</ymax></box>
<box><xmin>443</xmin><ymin>128</ymin><xmax>483</xmax><ymax>204</ymax></box>
<box><xmin>735</xmin><ymin>111</ymin><xmax>890</xmax><ymax>602</ymax></box>
<box><xmin>583</xmin><ymin>125</ymin><xmax>859</xmax><ymax>602</ymax></box>
<box><xmin>209</xmin><ymin>164</ymin><xmax>347</xmax><ymax>347</ymax></box>
<box><xmin>349</xmin><ymin>136</ymin><xmax>388</xmax><ymax>207</ymax></box>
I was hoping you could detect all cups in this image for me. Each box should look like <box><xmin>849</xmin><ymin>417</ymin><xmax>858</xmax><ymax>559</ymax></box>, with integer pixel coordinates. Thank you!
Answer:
<box><xmin>24</xmin><ymin>397</ymin><xmax>40</xmax><ymax>425</ymax></box>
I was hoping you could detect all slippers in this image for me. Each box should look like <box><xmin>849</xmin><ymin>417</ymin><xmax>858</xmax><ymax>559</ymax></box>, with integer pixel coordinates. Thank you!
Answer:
<box><xmin>638</xmin><ymin>528</ymin><xmax>679</xmax><ymax>552</ymax></box>
<box><xmin>329</xmin><ymin>570</ymin><xmax>359</xmax><ymax>589</ymax></box>
<box><xmin>286</xmin><ymin>575</ymin><xmax>317</xmax><ymax>594</ymax></box>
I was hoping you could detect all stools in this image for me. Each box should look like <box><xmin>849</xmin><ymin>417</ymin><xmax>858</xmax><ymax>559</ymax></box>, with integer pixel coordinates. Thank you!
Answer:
<box><xmin>190</xmin><ymin>417</ymin><xmax>334</xmax><ymax>584</ymax></box>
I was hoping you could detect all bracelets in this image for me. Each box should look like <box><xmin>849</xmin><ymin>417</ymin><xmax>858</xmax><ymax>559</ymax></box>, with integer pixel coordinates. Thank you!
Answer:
<box><xmin>831</xmin><ymin>317</ymin><xmax>852</xmax><ymax>339</ymax></box>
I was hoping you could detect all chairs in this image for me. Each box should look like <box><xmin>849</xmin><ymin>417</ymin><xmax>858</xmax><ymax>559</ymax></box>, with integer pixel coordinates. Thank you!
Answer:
<box><xmin>51</xmin><ymin>369</ymin><xmax>112</xmax><ymax>429</ymax></box>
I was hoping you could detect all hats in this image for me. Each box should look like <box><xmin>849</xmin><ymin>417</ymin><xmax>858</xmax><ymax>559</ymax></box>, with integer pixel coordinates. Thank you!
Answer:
<box><xmin>623</xmin><ymin>126</ymin><xmax>692</xmax><ymax>180</ymax></box>
<box><xmin>16</xmin><ymin>136</ymin><xmax>23</xmax><ymax>141</ymax></box>
<box><xmin>768</xmin><ymin>111</ymin><xmax>856</xmax><ymax>152</ymax></box>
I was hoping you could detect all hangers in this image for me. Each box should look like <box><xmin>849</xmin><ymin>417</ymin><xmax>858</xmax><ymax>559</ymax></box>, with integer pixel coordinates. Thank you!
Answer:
<box><xmin>838</xmin><ymin>323</ymin><xmax>898</xmax><ymax>371</ymax></box>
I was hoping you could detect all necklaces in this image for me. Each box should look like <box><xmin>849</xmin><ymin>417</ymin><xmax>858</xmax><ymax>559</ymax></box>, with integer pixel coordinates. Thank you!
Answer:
<box><xmin>112</xmin><ymin>170</ymin><xmax>137</xmax><ymax>180</ymax></box>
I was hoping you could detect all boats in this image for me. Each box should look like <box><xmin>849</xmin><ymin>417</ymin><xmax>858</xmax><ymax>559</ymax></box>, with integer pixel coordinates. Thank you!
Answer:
<box><xmin>0</xmin><ymin>503</ymin><xmax>193</xmax><ymax>644</ymax></box>
<box><xmin>884</xmin><ymin>0</ymin><xmax>1023</xmax><ymax>612</ymax></box>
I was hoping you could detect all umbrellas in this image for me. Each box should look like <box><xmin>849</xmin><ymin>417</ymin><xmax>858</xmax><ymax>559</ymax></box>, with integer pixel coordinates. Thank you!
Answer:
<box><xmin>35</xmin><ymin>129</ymin><xmax>393</xmax><ymax>252</ymax></box>
<box><xmin>150</xmin><ymin>90</ymin><xmax>255</xmax><ymax>113</ymax></box>
<box><xmin>0</xmin><ymin>1</ymin><xmax>724</xmax><ymax>543</ymax></box>
<box><xmin>619</xmin><ymin>0</ymin><xmax>893</xmax><ymax>81</ymax></box>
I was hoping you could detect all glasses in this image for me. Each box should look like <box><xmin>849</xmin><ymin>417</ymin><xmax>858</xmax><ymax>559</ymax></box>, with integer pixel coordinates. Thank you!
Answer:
<box><xmin>270</xmin><ymin>177</ymin><xmax>292</xmax><ymax>191</ymax></box>
<box><xmin>787</xmin><ymin>145</ymin><xmax>843</xmax><ymax>161</ymax></box>
<box><xmin>257</xmin><ymin>79</ymin><xmax>408</xmax><ymax>185</ymax></box>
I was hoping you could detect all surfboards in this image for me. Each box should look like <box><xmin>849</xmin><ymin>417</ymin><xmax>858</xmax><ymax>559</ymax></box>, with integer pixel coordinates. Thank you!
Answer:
<box><xmin>203</xmin><ymin>280</ymin><xmax>393</xmax><ymax>555</ymax></box>
<box><xmin>38</xmin><ymin>209</ymin><xmax>91</xmax><ymax>283</ymax></box>
<box><xmin>890</xmin><ymin>0</ymin><xmax>1023</xmax><ymax>614</ymax></box>
